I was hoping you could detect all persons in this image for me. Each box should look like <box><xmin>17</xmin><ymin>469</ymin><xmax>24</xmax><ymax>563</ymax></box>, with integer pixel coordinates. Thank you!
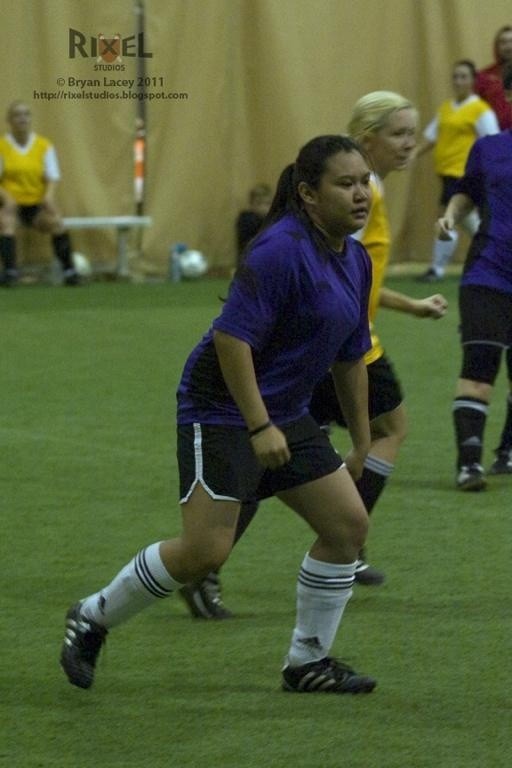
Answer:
<box><xmin>431</xmin><ymin>63</ymin><xmax>512</xmax><ymax>488</ymax></box>
<box><xmin>410</xmin><ymin>57</ymin><xmax>502</xmax><ymax>274</ymax></box>
<box><xmin>0</xmin><ymin>102</ymin><xmax>85</xmax><ymax>288</ymax></box>
<box><xmin>474</xmin><ymin>26</ymin><xmax>511</xmax><ymax>128</ymax></box>
<box><xmin>234</xmin><ymin>181</ymin><xmax>271</xmax><ymax>262</ymax></box>
<box><xmin>175</xmin><ymin>86</ymin><xmax>447</xmax><ymax>620</ymax></box>
<box><xmin>59</xmin><ymin>135</ymin><xmax>380</xmax><ymax>694</ymax></box>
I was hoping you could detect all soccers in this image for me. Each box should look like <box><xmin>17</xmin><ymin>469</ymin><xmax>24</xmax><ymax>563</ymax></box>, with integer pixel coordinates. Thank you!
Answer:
<box><xmin>179</xmin><ymin>251</ymin><xmax>207</xmax><ymax>278</ymax></box>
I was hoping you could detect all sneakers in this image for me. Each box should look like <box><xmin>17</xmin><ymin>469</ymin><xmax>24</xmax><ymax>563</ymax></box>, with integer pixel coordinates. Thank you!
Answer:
<box><xmin>353</xmin><ymin>558</ymin><xmax>384</xmax><ymax>585</ymax></box>
<box><xmin>58</xmin><ymin>595</ymin><xmax>110</xmax><ymax>688</ymax></box>
<box><xmin>63</xmin><ymin>265</ymin><xmax>80</xmax><ymax>285</ymax></box>
<box><xmin>178</xmin><ymin>572</ymin><xmax>234</xmax><ymax>619</ymax></box>
<box><xmin>417</xmin><ymin>267</ymin><xmax>440</xmax><ymax>283</ymax></box>
<box><xmin>488</xmin><ymin>458</ymin><xmax>511</xmax><ymax>475</ymax></box>
<box><xmin>280</xmin><ymin>654</ymin><xmax>377</xmax><ymax>695</ymax></box>
<box><xmin>455</xmin><ymin>460</ymin><xmax>490</xmax><ymax>491</ymax></box>
<box><xmin>1</xmin><ymin>267</ymin><xmax>19</xmax><ymax>286</ymax></box>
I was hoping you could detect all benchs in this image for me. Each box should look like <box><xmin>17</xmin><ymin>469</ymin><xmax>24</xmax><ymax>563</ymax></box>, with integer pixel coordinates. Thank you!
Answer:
<box><xmin>7</xmin><ymin>213</ymin><xmax>154</xmax><ymax>288</ymax></box>
